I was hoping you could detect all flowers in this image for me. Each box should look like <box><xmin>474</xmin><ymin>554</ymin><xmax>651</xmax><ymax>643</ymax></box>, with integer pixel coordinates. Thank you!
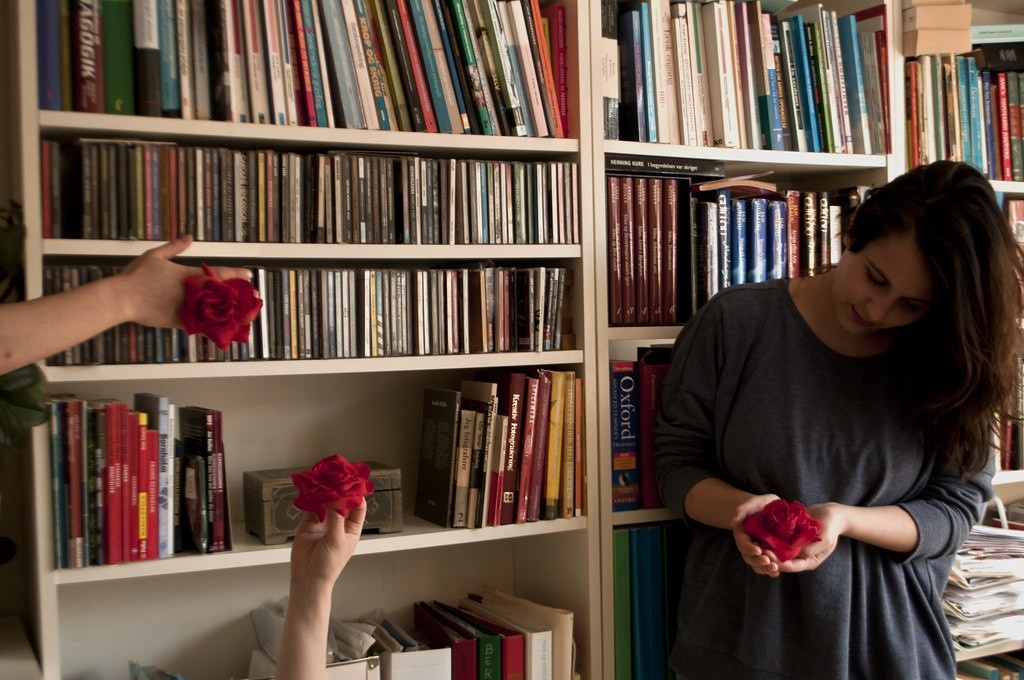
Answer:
<box><xmin>740</xmin><ymin>498</ymin><xmax>824</xmax><ymax>563</ymax></box>
<box><xmin>179</xmin><ymin>262</ymin><xmax>264</xmax><ymax>354</ymax></box>
<box><xmin>290</xmin><ymin>452</ymin><xmax>376</xmax><ymax>524</ymax></box>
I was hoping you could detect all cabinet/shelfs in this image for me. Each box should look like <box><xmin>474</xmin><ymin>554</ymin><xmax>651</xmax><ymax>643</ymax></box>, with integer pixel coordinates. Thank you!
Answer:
<box><xmin>588</xmin><ymin>0</ymin><xmax>900</xmax><ymax>680</ymax></box>
<box><xmin>895</xmin><ymin>24</ymin><xmax>1024</xmax><ymax>485</ymax></box>
<box><xmin>12</xmin><ymin>0</ymin><xmax>604</xmax><ymax>680</ymax></box>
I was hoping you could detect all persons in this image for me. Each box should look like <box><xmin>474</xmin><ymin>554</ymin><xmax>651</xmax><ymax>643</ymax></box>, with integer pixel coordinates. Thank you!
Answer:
<box><xmin>652</xmin><ymin>160</ymin><xmax>1024</xmax><ymax>680</ymax></box>
<box><xmin>0</xmin><ymin>233</ymin><xmax>264</xmax><ymax>381</ymax></box>
<box><xmin>274</xmin><ymin>491</ymin><xmax>366</xmax><ymax>680</ymax></box>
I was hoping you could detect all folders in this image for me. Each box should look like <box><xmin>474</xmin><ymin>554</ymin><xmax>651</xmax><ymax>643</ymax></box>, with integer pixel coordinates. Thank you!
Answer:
<box><xmin>254</xmin><ymin>583</ymin><xmax>577</xmax><ymax>680</ymax></box>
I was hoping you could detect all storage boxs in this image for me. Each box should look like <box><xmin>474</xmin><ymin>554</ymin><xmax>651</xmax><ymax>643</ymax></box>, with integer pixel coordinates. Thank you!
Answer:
<box><xmin>241</xmin><ymin>460</ymin><xmax>404</xmax><ymax>544</ymax></box>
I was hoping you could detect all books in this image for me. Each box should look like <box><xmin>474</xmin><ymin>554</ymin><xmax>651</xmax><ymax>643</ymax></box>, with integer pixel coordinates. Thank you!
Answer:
<box><xmin>36</xmin><ymin>0</ymin><xmax>1024</xmax><ymax>680</ymax></box>
